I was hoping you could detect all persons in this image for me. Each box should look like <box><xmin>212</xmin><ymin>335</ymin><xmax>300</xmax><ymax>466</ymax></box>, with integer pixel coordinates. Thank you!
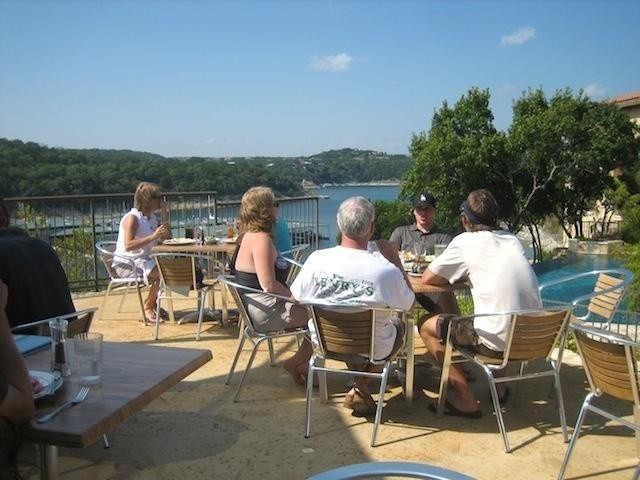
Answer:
<box><xmin>290</xmin><ymin>196</ymin><xmax>416</xmax><ymax>418</ymax></box>
<box><xmin>231</xmin><ymin>186</ymin><xmax>320</xmax><ymax>390</ymax></box>
<box><xmin>0</xmin><ymin>278</ymin><xmax>36</xmax><ymax>480</ymax></box>
<box><xmin>273</xmin><ymin>202</ymin><xmax>292</xmax><ymax>285</ymax></box>
<box><xmin>388</xmin><ymin>190</ymin><xmax>476</xmax><ymax>383</ymax></box>
<box><xmin>0</xmin><ymin>193</ymin><xmax>78</xmax><ymax>337</ymax></box>
<box><xmin>112</xmin><ymin>181</ymin><xmax>172</xmax><ymax>323</ymax></box>
<box><xmin>416</xmin><ymin>188</ymin><xmax>543</xmax><ymax>420</ymax></box>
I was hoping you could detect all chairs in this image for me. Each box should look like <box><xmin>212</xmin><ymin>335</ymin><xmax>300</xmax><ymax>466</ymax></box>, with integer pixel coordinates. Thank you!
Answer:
<box><xmin>10</xmin><ymin>307</ymin><xmax>110</xmax><ymax>450</ymax></box>
<box><xmin>216</xmin><ymin>274</ymin><xmax>311</xmax><ymax>401</ymax></box>
<box><xmin>558</xmin><ymin>322</ymin><xmax>640</xmax><ymax>480</ymax></box>
<box><xmin>306</xmin><ymin>461</ymin><xmax>474</xmax><ymax>480</ymax></box>
<box><xmin>96</xmin><ymin>235</ymin><xmax>310</xmax><ymax>342</ymax></box>
<box><xmin>433</xmin><ymin>305</ymin><xmax>582</xmax><ymax>455</ymax></box>
<box><xmin>297</xmin><ymin>299</ymin><xmax>414</xmax><ymax>448</ymax></box>
<box><xmin>536</xmin><ymin>269</ymin><xmax>633</xmax><ymax>389</ymax></box>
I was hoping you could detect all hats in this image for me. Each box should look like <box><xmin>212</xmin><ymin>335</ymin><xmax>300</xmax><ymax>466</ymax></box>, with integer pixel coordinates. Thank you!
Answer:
<box><xmin>411</xmin><ymin>192</ymin><xmax>435</xmax><ymax>212</ymax></box>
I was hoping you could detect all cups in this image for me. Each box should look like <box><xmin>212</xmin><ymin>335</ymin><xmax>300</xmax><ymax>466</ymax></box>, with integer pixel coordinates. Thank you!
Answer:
<box><xmin>414</xmin><ymin>243</ymin><xmax>425</xmax><ymax>264</ymax></box>
<box><xmin>72</xmin><ymin>331</ymin><xmax>104</xmax><ymax>383</ymax></box>
<box><xmin>434</xmin><ymin>244</ymin><xmax>446</xmax><ymax>258</ymax></box>
<box><xmin>184</xmin><ymin>223</ymin><xmax>194</xmax><ymax>238</ymax></box>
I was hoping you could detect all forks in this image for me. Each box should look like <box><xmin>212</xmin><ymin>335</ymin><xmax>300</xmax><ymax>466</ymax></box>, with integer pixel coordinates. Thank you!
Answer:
<box><xmin>38</xmin><ymin>385</ymin><xmax>90</xmax><ymax>424</ymax></box>
<box><xmin>46</xmin><ymin>369</ymin><xmax>61</xmax><ymax>396</ymax></box>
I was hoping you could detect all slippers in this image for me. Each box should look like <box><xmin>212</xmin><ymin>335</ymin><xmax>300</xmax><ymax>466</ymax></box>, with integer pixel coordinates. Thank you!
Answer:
<box><xmin>429</xmin><ymin>397</ymin><xmax>482</xmax><ymax>420</ymax></box>
<box><xmin>498</xmin><ymin>385</ymin><xmax>510</xmax><ymax>403</ymax></box>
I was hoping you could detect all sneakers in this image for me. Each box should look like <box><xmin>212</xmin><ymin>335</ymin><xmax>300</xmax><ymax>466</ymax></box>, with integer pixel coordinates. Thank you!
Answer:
<box><xmin>343</xmin><ymin>391</ymin><xmax>376</xmax><ymax>418</ymax></box>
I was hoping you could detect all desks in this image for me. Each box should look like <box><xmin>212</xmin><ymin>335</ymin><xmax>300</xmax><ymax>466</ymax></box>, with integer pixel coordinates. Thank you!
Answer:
<box><xmin>397</xmin><ymin>271</ymin><xmax>468</xmax><ymax>384</ymax></box>
<box><xmin>15</xmin><ymin>337</ymin><xmax>214</xmax><ymax>480</ymax></box>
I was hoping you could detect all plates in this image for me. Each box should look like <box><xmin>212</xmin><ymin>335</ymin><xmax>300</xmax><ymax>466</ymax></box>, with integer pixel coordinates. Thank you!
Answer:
<box><xmin>26</xmin><ymin>368</ymin><xmax>64</xmax><ymax>400</ymax></box>
<box><xmin>222</xmin><ymin>237</ymin><xmax>237</xmax><ymax>244</ymax></box>
<box><xmin>403</xmin><ymin>254</ymin><xmax>437</xmax><ymax>277</ymax></box>
<box><xmin>204</xmin><ymin>237</ymin><xmax>220</xmax><ymax>244</ymax></box>
<box><xmin>160</xmin><ymin>238</ymin><xmax>195</xmax><ymax>244</ymax></box>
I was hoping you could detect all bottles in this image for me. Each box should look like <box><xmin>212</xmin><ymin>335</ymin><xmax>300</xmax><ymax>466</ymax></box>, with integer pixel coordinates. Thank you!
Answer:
<box><xmin>195</xmin><ymin>235</ymin><xmax>200</xmax><ymax>245</ymax></box>
<box><xmin>48</xmin><ymin>316</ymin><xmax>70</xmax><ymax>375</ymax></box>
